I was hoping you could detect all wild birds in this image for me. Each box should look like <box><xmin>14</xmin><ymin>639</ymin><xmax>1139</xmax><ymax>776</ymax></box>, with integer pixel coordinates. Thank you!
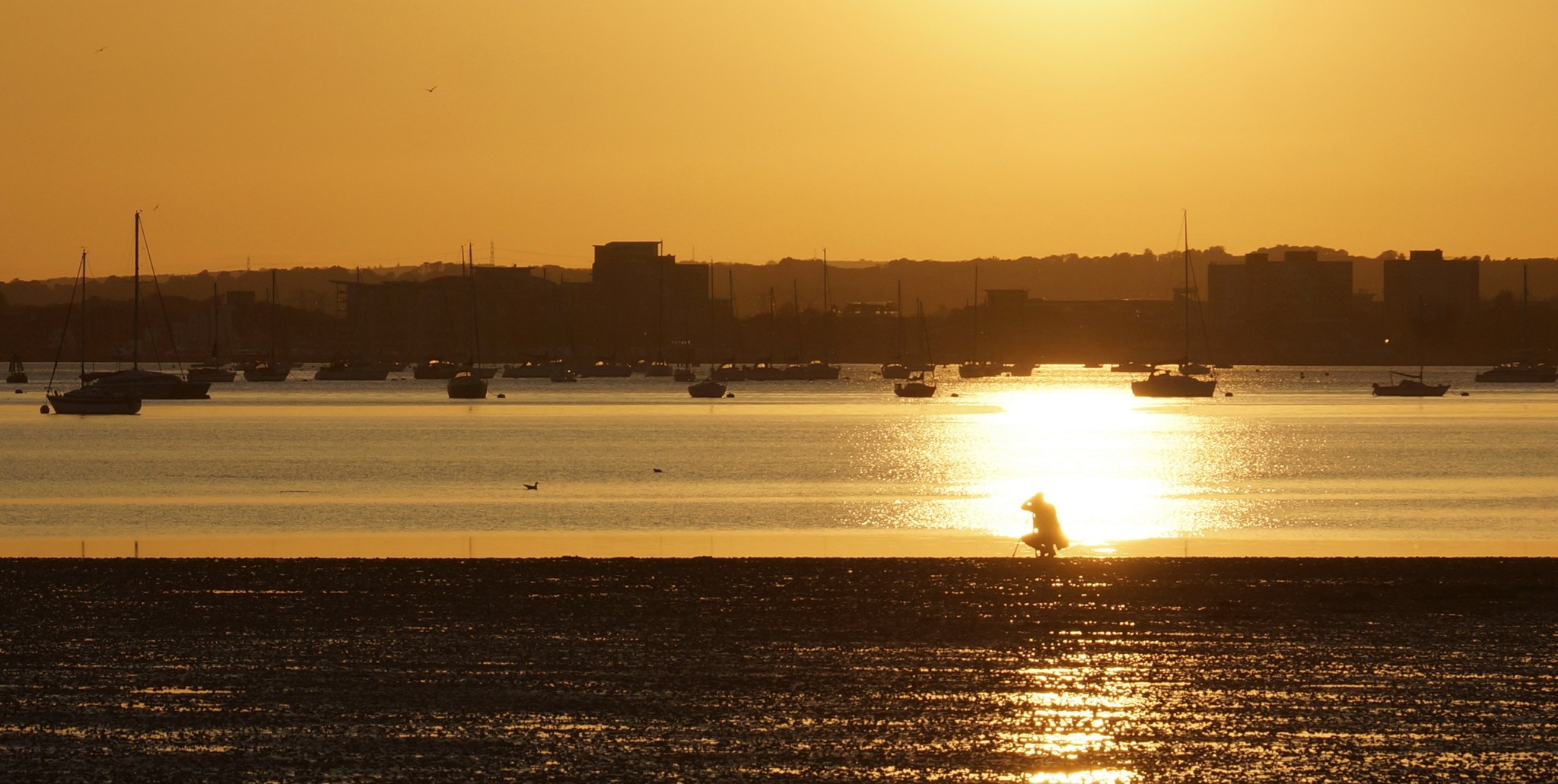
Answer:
<box><xmin>653</xmin><ymin>468</ymin><xmax>662</xmax><ymax>472</ymax></box>
<box><xmin>523</xmin><ymin>482</ymin><xmax>539</xmax><ymax>490</ymax></box>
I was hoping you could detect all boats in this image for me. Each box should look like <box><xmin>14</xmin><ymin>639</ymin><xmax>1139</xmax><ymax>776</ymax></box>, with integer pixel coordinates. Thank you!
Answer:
<box><xmin>447</xmin><ymin>375</ymin><xmax>491</xmax><ymax>399</ymax></box>
<box><xmin>1371</xmin><ymin>363</ymin><xmax>1452</xmax><ymax>397</ymax></box>
<box><xmin>1082</xmin><ymin>362</ymin><xmax>1103</xmax><ymax>368</ymax></box>
<box><xmin>1110</xmin><ymin>358</ymin><xmax>1155</xmax><ymax>372</ymax></box>
<box><xmin>1179</xmin><ymin>360</ymin><xmax>1211</xmax><ymax>375</ymax></box>
<box><xmin>687</xmin><ymin>375</ymin><xmax>728</xmax><ymax>399</ymax></box>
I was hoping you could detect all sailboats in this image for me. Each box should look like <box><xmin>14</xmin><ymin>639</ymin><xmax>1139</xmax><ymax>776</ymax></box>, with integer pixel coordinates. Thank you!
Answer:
<box><xmin>959</xmin><ymin>265</ymin><xmax>1035</xmax><ymax>378</ymax></box>
<box><xmin>881</xmin><ymin>280</ymin><xmax>912</xmax><ymax>380</ymax></box>
<box><xmin>243</xmin><ymin>269</ymin><xmax>292</xmax><ymax>383</ymax></box>
<box><xmin>904</xmin><ymin>300</ymin><xmax>935</xmax><ymax>371</ymax></box>
<box><xmin>190</xmin><ymin>196</ymin><xmax>845</xmax><ymax>381</ymax></box>
<box><xmin>43</xmin><ymin>245</ymin><xmax>143</xmax><ymax>415</ymax></box>
<box><xmin>187</xmin><ymin>282</ymin><xmax>238</xmax><ymax>385</ymax></box>
<box><xmin>1130</xmin><ymin>209</ymin><xmax>1218</xmax><ymax>397</ymax></box>
<box><xmin>6</xmin><ymin>352</ymin><xmax>29</xmax><ymax>383</ymax></box>
<box><xmin>1472</xmin><ymin>263</ymin><xmax>1558</xmax><ymax>383</ymax></box>
<box><xmin>894</xmin><ymin>299</ymin><xmax>937</xmax><ymax>398</ymax></box>
<box><xmin>75</xmin><ymin>209</ymin><xmax>212</xmax><ymax>400</ymax></box>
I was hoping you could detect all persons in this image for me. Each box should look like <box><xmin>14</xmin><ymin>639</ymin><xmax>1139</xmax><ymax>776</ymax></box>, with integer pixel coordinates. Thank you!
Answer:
<box><xmin>1021</xmin><ymin>493</ymin><xmax>1067</xmax><ymax>557</ymax></box>
